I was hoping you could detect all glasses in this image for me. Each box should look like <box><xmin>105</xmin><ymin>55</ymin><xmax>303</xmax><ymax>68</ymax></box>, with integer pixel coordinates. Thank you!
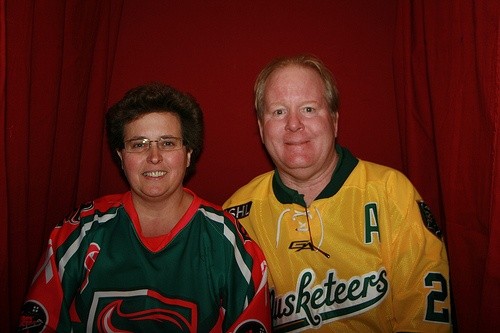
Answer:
<box><xmin>121</xmin><ymin>136</ymin><xmax>188</xmax><ymax>153</ymax></box>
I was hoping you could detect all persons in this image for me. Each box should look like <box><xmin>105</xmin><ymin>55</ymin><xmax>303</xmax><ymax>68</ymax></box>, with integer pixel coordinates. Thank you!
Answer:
<box><xmin>19</xmin><ymin>82</ymin><xmax>273</xmax><ymax>333</ymax></box>
<box><xmin>221</xmin><ymin>55</ymin><xmax>451</xmax><ymax>331</ymax></box>
